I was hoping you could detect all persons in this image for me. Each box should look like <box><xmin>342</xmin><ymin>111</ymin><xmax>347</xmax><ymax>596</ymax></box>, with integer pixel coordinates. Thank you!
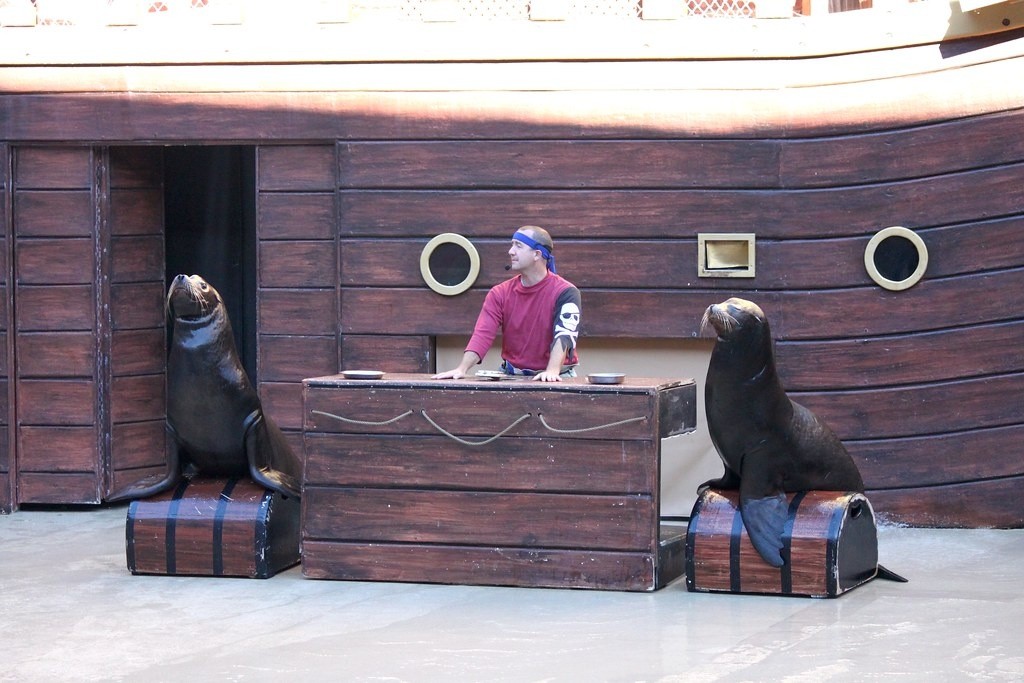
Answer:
<box><xmin>430</xmin><ymin>225</ymin><xmax>582</xmax><ymax>381</ymax></box>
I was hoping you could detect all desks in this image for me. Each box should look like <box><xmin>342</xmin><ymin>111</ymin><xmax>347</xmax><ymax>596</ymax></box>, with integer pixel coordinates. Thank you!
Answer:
<box><xmin>300</xmin><ymin>374</ymin><xmax>699</xmax><ymax>591</ymax></box>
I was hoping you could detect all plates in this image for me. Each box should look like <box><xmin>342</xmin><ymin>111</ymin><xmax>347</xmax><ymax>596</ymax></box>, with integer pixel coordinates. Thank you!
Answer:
<box><xmin>587</xmin><ymin>374</ymin><xmax>625</xmax><ymax>384</ymax></box>
<box><xmin>340</xmin><ymin>370</ymin><xmax>386</xmax><ymax>379</ymax></box>
